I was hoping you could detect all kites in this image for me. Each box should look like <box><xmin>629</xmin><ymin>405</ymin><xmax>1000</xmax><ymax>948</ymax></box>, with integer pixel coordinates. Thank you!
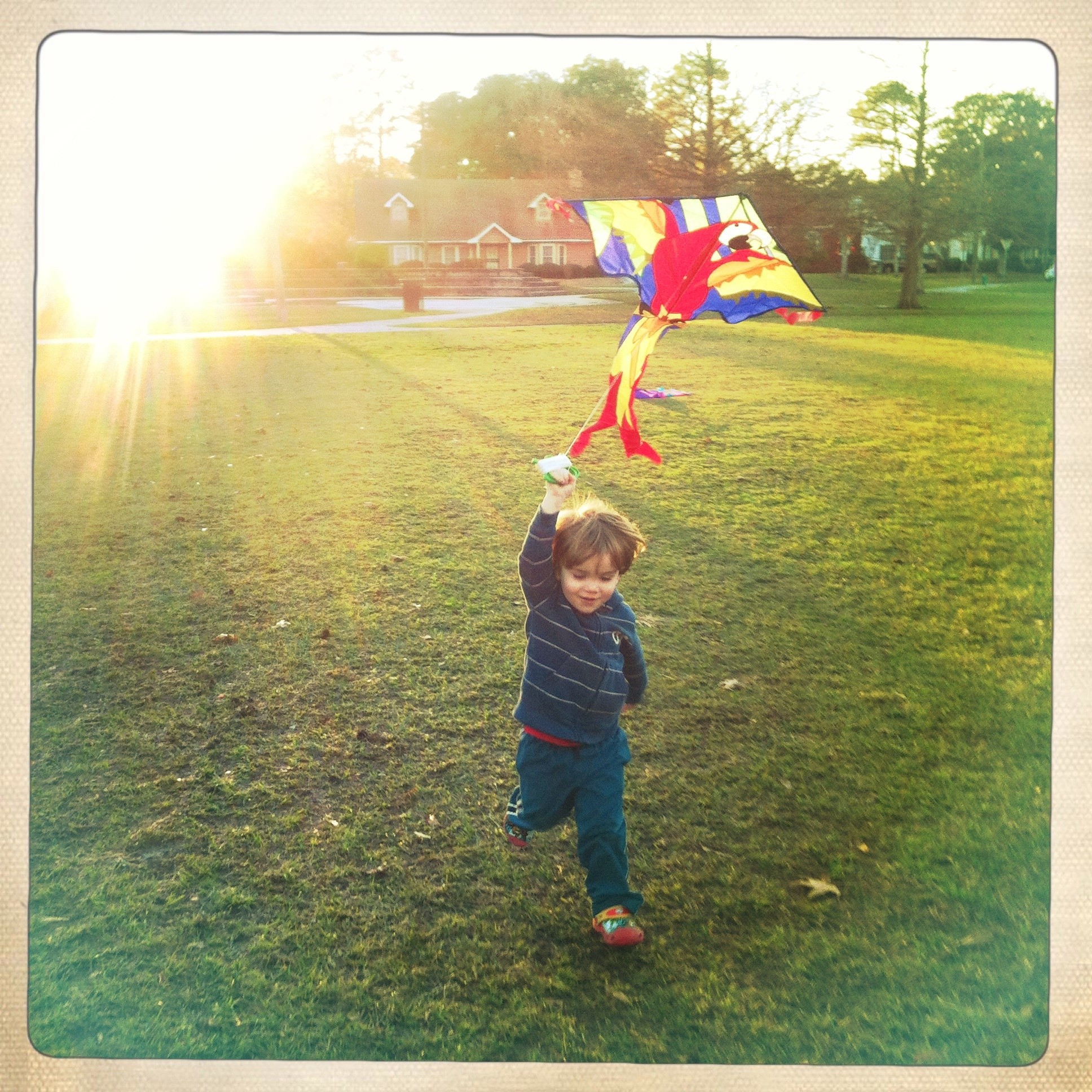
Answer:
<box><xmin>553</xmin><ymin>193</ymin><xmax>827</xmax><ymax>467</ymax></box>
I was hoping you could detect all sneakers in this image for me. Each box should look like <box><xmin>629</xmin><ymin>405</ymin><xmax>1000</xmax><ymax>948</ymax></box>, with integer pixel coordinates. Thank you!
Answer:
<box><xmin>592</xmin><ymin>904</ymin><xmax>644</xmax><ymax>946</ymax></box>
<box><xmin>503</xmin><ymin>786</ymin><xmax>535</xmax><ymax>847</ymax></box>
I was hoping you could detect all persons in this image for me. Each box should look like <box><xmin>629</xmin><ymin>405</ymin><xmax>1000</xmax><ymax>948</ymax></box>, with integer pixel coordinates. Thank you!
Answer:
<box><xmin>504</xmin><ymin>466</ymin><xmax>650</xmax><ymax>947</ymax></box>
